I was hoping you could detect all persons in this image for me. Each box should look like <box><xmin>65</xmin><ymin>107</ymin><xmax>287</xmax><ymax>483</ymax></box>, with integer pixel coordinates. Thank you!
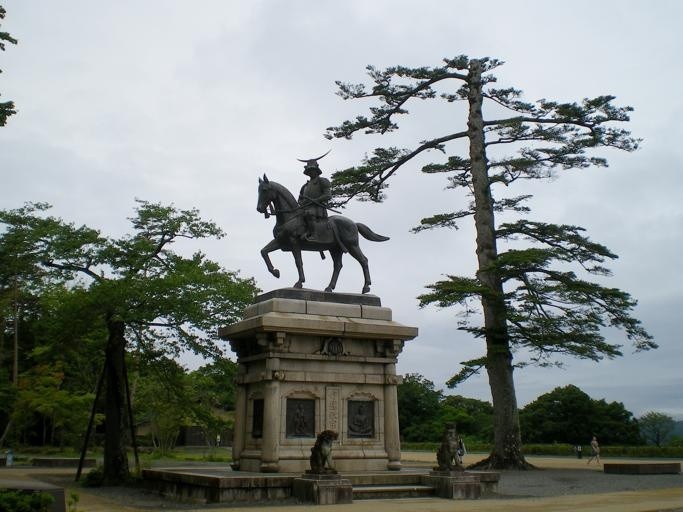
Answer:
<box><xmin>458</xmin><ymin>439</ymin><xmax>467</xmax><ymax>464</ymax></box>
<box><xmin>348</xmin><ymin>405</ymin><xmax>372</xmax><ymax>433</ymax></box>
<box><xmin>587</xmin><ymin>436</ymin><xmax>601</xmax><ymax>464</ymax></box>
<box><xmin>297</xmin><ymin>159</ymin><xmax>332</xmax><ymax>241</ymax></box>
<box><xmin>292</xmin><ymin>403</ymin><xmax>307</xmax><ymax>435</ymax></box>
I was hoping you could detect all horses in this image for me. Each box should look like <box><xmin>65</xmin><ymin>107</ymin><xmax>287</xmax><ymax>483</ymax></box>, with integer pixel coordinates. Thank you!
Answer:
<box><xmin>254</xmin><ymin>172</ymin><xmax>391</xmax><ymax>293</ymax></box>
